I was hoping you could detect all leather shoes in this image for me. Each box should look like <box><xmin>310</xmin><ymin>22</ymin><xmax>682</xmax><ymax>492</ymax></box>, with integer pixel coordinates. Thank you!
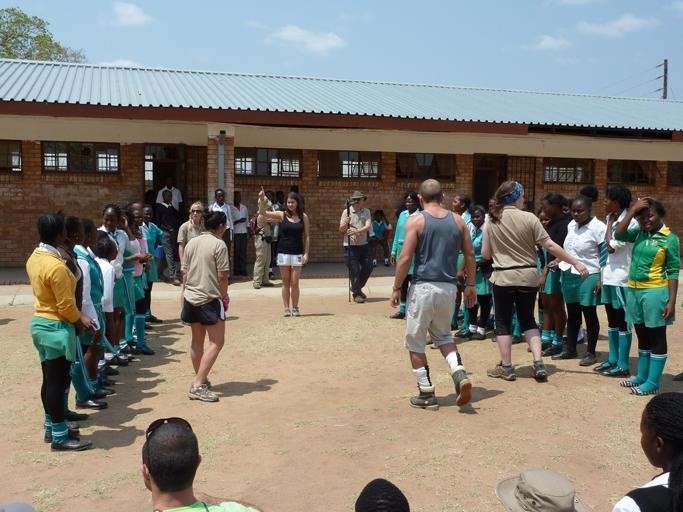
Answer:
<box><xmin>45</xmin><ymin>316</ymin><xmax>162</xmax><ymax>451</ymax></box>
<box><xmin>390</xmin><ymin>312</ymin><xmax>631</xmax><ymax>377</ymax></box>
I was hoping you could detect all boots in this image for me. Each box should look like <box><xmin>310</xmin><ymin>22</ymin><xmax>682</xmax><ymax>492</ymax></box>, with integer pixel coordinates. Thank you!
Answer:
<box><xmin>451</xmin><ymin>366</ymin><xmax>472</xmax><ymax>406</ymax></box>
<box><xmin>409</xmin><ymin>382</ymin><xmax>439</xmax><ymax>411</ymax></box>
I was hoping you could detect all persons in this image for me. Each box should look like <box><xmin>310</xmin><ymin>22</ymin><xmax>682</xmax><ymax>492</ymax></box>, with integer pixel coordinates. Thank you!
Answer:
<box><xmin>367</xmin><ymin>210</ymin><xmax>392</xmax><ymax>267</ymax></box>
<box><xmin>611</xmin><ymin>391</ymin><xmax>683</xmax><ymax>512</ymax></box>
<box><xmin>252</xmin><ymin>185</ymin><xmax>310</xmax><ymax>316</ymax></box>
<box><xmin>26</xmin><ymin>203</ymin><xmax>164</xmax><ymax>450</ymax></box>
<box><xmin>340</xmin><ymin>190</ymin><xmax>372</xmax><ymax>303</ymax></box>
<box><xmin>389</xmin><ymin>179</ymin><xmax>679</xmax><ymax>411</ymax></box>
<box><xmin>147</xmin><ymin>176</ymin><xmax>249</xmax><ymax>325</ymax></box>
<box><xmin>180</xmin><ymin>209</ymin><xmax>231</xmax><ymax>402</ymax></box>
<box><xmin>140</xmin><ymin>417</ymin><xmax>263</xmax><ymax>511</ymax></box>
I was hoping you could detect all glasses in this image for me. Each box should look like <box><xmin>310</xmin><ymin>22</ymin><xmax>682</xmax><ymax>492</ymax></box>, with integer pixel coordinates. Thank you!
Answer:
<box><xmin>146</xmin><ymin>418</ymin><xmax>192</xmax><ymax>474</ymax></box>
<box><xmin>353</xmin><ymin>198</ymin><xmax>361</xmax><ymax>201</ymax></box>
<box><xmin>191</xmin><ymin>209</ymin><xmax>202</xmax><ymax>212</ymax></box>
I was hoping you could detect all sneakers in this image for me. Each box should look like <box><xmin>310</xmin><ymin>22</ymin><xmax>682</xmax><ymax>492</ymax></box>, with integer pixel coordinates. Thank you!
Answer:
<box><xmin>487</xmin><ymin>361</ymin><xmax>516</xmax><ymax>381</ymax></box>
<box><xmin>188</xmin><ymin>384</ymin><xmax>219</xmax><ymax>401</ymax></box>
<box><xmin>350</xmin><ymin>286</ymin><xmax>366</xmax><ymax>303</ymax></box>
<box><xmin>631</xmin><ymin>386</ymin><xmax>660</xmax><ymax>396</ymax></box>
<box><xmin>284</xmin><ymin>309</ymin><xmax>291</xmax><ymax>317</ymax></box>
<box><xmin>619</xmin><ymin>376</ymin><xmax>647</xmax><ymax>387</ymax></box>
<box><xmin>171</xmin><ymin>278</ymin><xmax>180</xmax><ymax>285</ymax></box>
<box><xmin>532</xmin><ymin>364</ymin><xmax>547</xmax><ymax>379</ymax></box>
<box><xmin>252</xmin><ymin>281</ymin><xmax>274</xmax><ymax>289</ymax></box>
<box><xmin>292</xmin><ymin>306</ymin><xmax>299</xmax><ymax>316</ymax></box>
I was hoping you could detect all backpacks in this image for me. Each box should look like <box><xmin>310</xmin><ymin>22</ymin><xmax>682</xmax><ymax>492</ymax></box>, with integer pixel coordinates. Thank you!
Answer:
<box><xmin>247</xmin><ymin>213</ymin><xmax>263</xmax><ymax>240</ymax></box>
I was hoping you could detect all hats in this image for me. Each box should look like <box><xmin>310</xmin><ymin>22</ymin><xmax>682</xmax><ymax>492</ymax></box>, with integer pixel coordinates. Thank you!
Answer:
<box><xmin>352</xmin><ymin>191</ymin><xmax>366</xmax><ymax>201</ymax></box>
